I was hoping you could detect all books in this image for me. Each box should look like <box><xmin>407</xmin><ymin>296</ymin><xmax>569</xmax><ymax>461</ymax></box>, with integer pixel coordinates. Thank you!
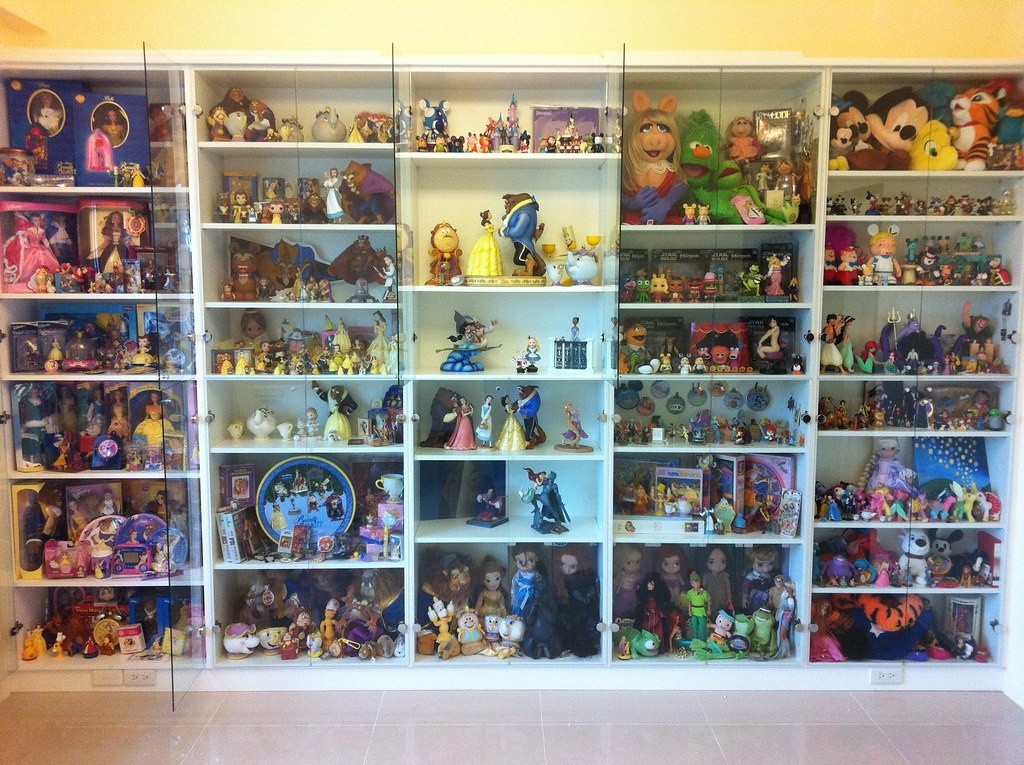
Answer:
<box><xmin>977</xmin><ymin>530</ymin><xmax>1002</xmax><ymax>587</ymax></box>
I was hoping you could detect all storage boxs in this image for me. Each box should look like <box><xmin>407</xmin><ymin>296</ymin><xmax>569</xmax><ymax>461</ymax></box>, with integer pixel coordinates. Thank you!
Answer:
<box><xmin>5</xmin><ymin>77</ymin><xmax>151</xmax><ymax>186</ymax></box>
<box><xmin>216</xmin><ymin>506</ymin><xmax>262</xmax><ymax>563</ymax></box>
<box><xmin>0</xmin><ymin>194</ymin><xmax>151</xmax><ymax>293</ymax></box>
<box><xmin>613</xmin><ymin>454</ymin><xmax>798</xmax><ymax>537</ymax></box>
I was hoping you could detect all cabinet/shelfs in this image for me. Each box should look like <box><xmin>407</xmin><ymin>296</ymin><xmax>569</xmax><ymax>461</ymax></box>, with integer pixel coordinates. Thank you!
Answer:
<box><xmin>0</xmin><ymin>49</ymin><xmax>1024</xmax><ymax>698</ymax></box>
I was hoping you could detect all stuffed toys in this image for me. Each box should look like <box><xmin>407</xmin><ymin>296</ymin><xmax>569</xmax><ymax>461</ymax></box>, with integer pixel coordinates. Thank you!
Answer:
<box><xmin>620</xmin><ymin>89</ymin><xmax>800</xmax><ymax>224</ymax></box>
<box><xmin>810</xmin><ymin>528</ymin><xmax>972</xmax><ymax>663</ymax></box>
<box><xmin>828</xmin><ymin>79</ymin><xmax>1024</xmax><ymax>170</ymax></box>
<box><xmin>824</xmin><ymin>191</ymin><xmax>1016</xmax><ymax>286</ymax></box>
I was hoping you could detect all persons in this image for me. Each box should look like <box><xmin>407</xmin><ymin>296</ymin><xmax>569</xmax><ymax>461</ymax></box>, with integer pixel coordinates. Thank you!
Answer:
<box><xmin>620</xmin><ymin>266</ymin><xmax>721</xmax><ymax>304</ymax></box>
<box><xmin>875</xmin><ymin>562</ymin><xmax>889</xmax><ymax>588</ymax></box>
<box><xmin>819</xmin><ymin>299</ymin><xmax>993</xmax><ymax>432</ymax></box>
<box><xmin>205</xmin><ymin>87</ymin><xmax>600</xmax><ymax>661</ymax></box>
<box><xmin>17</xmin><ymin>94</ymin><xmax>201</xmax><ymax>661</ymax></box>
<box><xmin>612</xmin><ymin>316</ymin><xmax>800</xmax><ymax>659</ymax></box>
<box><xmin>764</xmin><ymin>253</ymin><xmax>799</xmax><ymax>302</ymax></box>
<box><xmin>809</xmin><ymin>598</ymin><xmax>848</xmax><ymax>662</ymax></box>
<box><xmin>827</xmin><ymin>437</ymin><xmax>917</xmax><ymax>524</ymax></box>
